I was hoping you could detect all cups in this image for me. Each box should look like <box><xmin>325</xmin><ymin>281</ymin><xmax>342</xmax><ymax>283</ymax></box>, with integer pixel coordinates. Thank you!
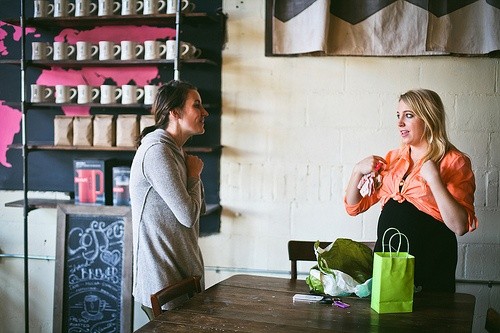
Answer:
<box><xmin>100</xmin><ymin>85</ymin><xmax>122</xmax><ymax>105</ymax></box>
<box><xmin>122</xmin><ymin>84</ymin><xmax>144</xmax><ymax>105</ymax></box>
<box><xmin>75</xmin><ymin>41</ymin><xmax>99</xmax><ymax>61</ymax></box>
<box><xmin>98</xmin><ymin>41</ymin><xmax>121</xmax><ymax>61</ymax></box>
<box><xmin>30</xmin><ymin>84</ymin><xmax>53</xmax><ymax>103</ymax></box>
<box><xmin>53</xmin><ymin>42</ymin><xmax>75</xmax><ymax>61</ymax></box>
<box><xmin>32</xmin><ymin>42</ymin><xmax>53</xmax><ymax>60</ymax></box>
<box><xmin>33</xmin><ymin>0</ymin><xmax>189</xmax><ymax>18</ymax></box>
<box><xmin>77</xmin><ymin>85</ymin><xmax>99</xmax><ymax>104</ymax></box>
<box><xmin>55</xmin><ymin>85</ymin><xmax>77</xmax><ymax>103</ymax></box>
<box><xmin>145</xmin><ymin>85</ymin><xmax>162</xmax><ymax>105</ymax></box>
<box><xmin>183</xmin><ymin>42</ymin><xmax>197</xmax><ymax>59</ymax></box>
<box><xmin>121</xmin><ymin>41</ymin><xmax>144</xmax><ymax>60</ymax></box>
<box><xmin>144</xmin><ymin>40</ymin><xmax>188</xmax><ymax>61</ymax></box>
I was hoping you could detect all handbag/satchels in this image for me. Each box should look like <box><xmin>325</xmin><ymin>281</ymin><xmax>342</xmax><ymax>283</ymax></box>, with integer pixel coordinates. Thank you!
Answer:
<box><xmin>370</xmin><ymin>227</ymin><xmax>416</xmax><ymax>314</ymax></box>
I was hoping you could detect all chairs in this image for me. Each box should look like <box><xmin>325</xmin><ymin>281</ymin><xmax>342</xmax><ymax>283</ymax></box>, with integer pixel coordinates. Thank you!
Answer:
<box><xmin>151</xmin><ymin>276</ymin><xmax>202</xmax><ymax>316</ymax></box>
<box><xmin>288</xmin><ymin>241</ymin><xmax>376</xmax><ymax>280</ymax></box>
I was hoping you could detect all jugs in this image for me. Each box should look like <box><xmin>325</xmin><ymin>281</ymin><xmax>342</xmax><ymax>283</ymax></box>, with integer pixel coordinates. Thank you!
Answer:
<box><xmin>76</xmin><ymin>168</ymin><xmax>104</xmax><ymax>203</ymax></box>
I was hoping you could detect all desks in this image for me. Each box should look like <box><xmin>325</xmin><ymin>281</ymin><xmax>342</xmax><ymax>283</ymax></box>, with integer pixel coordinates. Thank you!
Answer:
<box><xmin>134</xmin><ymin>274</ymin><xmax>476</xmax><ymax>333</ymax></box>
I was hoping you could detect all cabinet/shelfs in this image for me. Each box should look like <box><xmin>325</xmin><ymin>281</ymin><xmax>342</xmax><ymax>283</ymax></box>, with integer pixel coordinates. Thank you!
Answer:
<box><xmin>0</xmin><ymin>0</ymin><xmax>224</xmax><ymax>333</ymax></box>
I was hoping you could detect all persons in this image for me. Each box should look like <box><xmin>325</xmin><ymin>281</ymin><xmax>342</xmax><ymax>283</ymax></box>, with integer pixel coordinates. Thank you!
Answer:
<box><xmin>129</xmin><ymin>80</ymin><xmax>207</xmax><ymax>321</ymax></box>
<box><xmin>345</xmin><ymin>89</ymin><xmax>479</xmax><ymax>293</ymax></box>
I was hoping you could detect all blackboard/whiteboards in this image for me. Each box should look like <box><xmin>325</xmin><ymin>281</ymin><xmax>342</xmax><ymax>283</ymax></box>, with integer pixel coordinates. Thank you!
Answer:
<box><xmin>53</xmin><ymin>202</ymin><xmax>133</xmax><ymax>333</ymax></box>
